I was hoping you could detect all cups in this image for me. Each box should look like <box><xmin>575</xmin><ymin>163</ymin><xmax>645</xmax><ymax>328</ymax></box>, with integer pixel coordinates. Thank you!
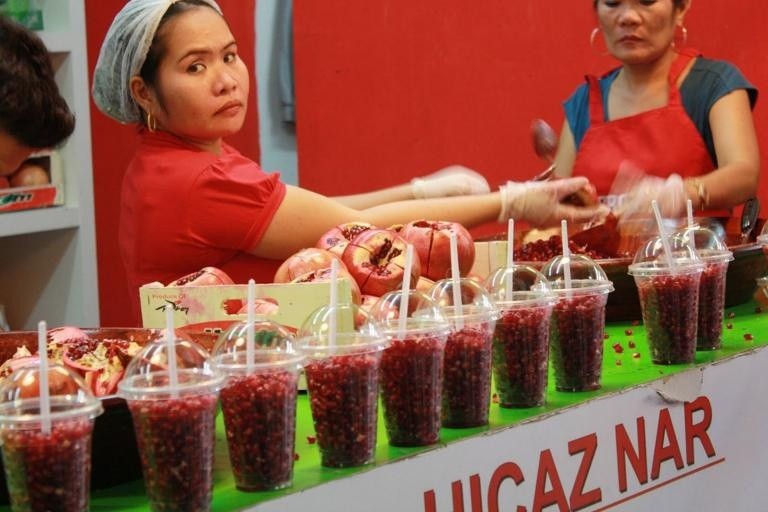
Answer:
<box><xmin>626</xmin><ymin>225</ymin><xmax>738</xmax><ymax>365</ymax></box>
<box><xmin>292</xmin><ymin>300</ymin><xmax>394</xmax><ymax>470</ymax></box>
<box><xmin>528</xmin><ymin>252</ymin><xmax>617</xmax><ymax>394</ymax></box>
<box><xmin>359</xmin><ymin>287</ymin><xmax>453</xmax><ymax>450</ymax></box>
<box><xmin>410</xmin><ymin>269</ymin><xmax>505</xmax><ymax>430</ymax></box>
<box><xmin>472</xmin><ymin>262</ymin><xmax>562</xmax><ymax>410</ymax></box>
<box><xmin>202</xmin><ymin>314</ymin><xmax>313</xmax><ymax>494</ymax></box>
<box><xmin>0</xmin><ymin>359</ymin><xmax>107</xmax><ymax>512</ymax></box>
<box><xmin>114</xmin><ymin>327</ymin><xmax>231</xmax><ymax>510</ymax></box>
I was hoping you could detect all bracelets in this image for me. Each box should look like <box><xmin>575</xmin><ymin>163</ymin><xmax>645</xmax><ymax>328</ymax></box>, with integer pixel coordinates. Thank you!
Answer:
<box><xmin>687</xmin><ymin>177</ymin><xmax>708</xmax><ymax>214</ymax></box>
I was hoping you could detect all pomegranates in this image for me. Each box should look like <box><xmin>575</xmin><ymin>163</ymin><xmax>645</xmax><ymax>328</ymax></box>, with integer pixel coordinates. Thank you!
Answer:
<box><xmin>0</xmin><ymin>218</ymin><xmax>490</xmax><ymax>401</ymax></box>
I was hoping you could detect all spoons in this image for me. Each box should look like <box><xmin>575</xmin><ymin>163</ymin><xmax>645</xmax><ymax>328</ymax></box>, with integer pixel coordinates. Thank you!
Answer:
<box><xmin>530</xmin><ymin>116</ymin><xmax>558</xmax><ymax>173</ymax></box>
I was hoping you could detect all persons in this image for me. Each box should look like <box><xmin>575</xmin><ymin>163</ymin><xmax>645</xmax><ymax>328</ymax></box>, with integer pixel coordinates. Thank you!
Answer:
<box><xmin>91</xmin><ymin>0</ymin><xmax>611</xmax><ymax>328</ymax></box>
<box><xmin>520</xmin><ymin>0</ymin><xmax>761</xmax><ymax>247</ymax></box>
<box><xmin>0</xmin><ymin>12</ymin><xmax>75</xmax><ymax>178</ymax></box>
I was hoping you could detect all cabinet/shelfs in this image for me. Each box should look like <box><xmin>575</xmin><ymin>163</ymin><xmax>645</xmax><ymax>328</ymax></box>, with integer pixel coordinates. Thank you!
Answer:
<box><xmin>0</xmin><ymin>0</ymin><xmax>100</xmax><ymax>332</ymax></box>
